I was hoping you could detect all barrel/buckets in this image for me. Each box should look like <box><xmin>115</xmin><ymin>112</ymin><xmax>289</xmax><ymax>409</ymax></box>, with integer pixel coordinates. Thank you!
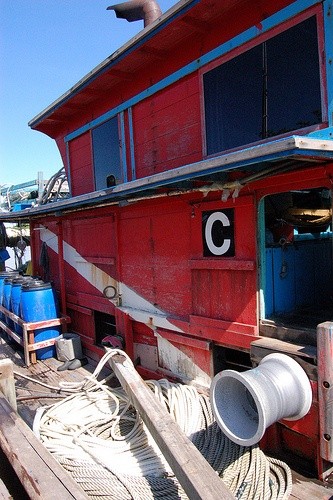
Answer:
<box><xmin>12</xmin><ymin>276</ymin><xmax>40</xmax><ymax>338</ymax></box>
<box><xmin>22</xmin><ymin>280</ymin><xmax>62</xmax><ymax>360</ymax></box>
<box><xmin>4</xmin><ymin>275</ymin><xmax>34</xmax><ymax>340</ymax></box>
<box><xmin>0</xmin><ymin>271</ymin><xmax>21</xmax><ymax>320</ymax></box>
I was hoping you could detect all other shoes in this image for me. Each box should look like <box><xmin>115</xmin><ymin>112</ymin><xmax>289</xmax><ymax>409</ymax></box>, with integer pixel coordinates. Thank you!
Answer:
<box><xmin>68</xmin><ymin>357</ymin><xmax>88</xmax><ymax>370</ymax></box>
<box><xmin>56</xmin><ymin>359</ymin><xmax>73</xmax><ymax>371</ymax></box>
